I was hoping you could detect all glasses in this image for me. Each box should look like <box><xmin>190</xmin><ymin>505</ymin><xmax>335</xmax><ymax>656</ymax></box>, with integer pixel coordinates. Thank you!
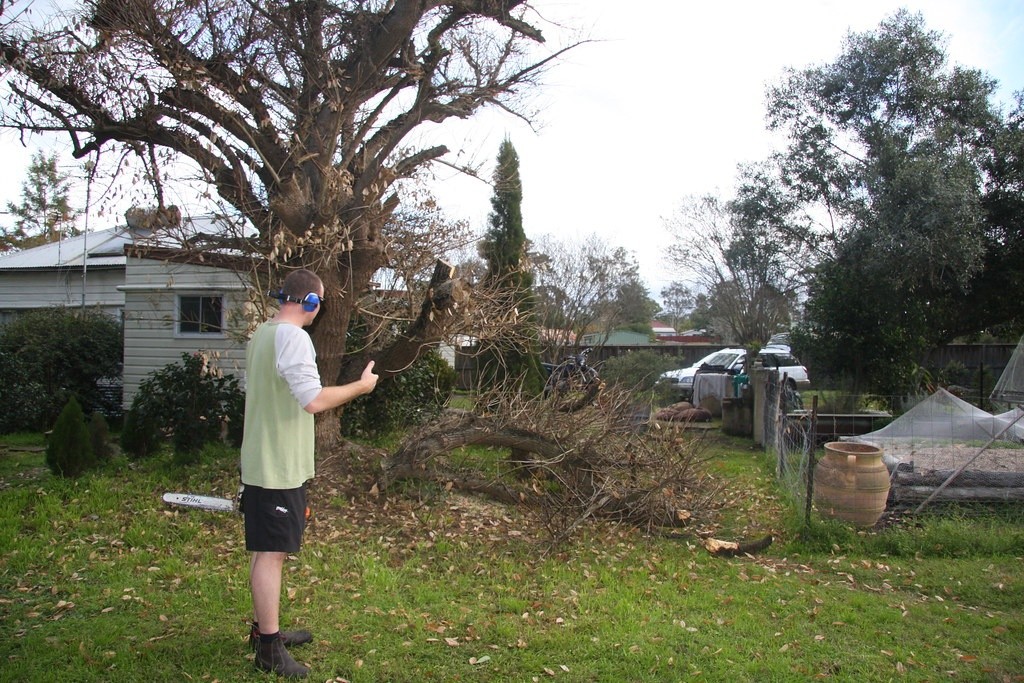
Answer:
<box><xmin>318</xmin><ymin>296</ymin><xmax>325</xmax><ymax>305</ymax></box>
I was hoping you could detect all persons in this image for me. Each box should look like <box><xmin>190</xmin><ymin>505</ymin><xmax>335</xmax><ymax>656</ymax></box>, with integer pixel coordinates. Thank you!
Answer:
<box><xmin>240</xmin><ymin>269</ymin><xmax>379</xmax><ymax>680</ymax></box>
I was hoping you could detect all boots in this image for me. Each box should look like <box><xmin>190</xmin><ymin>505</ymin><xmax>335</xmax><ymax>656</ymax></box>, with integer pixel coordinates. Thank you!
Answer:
<box><xmin>249</xmin><ymin>622</ymin><xmax>313</xmax><ymax>651</ymax></box>
<box><xmin>254</xmin><ymin>632</ymin><xmax>310</xmax><ymax>679</ymax></box>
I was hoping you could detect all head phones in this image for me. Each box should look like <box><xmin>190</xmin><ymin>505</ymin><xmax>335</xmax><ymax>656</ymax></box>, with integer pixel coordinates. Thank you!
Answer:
<box><xmin>268</xmin><ymin>289</ymin><xmax>319</xmax><ymax>312</ymax></box>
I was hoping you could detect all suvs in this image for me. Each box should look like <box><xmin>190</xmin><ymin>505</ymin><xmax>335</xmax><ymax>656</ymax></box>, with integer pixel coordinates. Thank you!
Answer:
<box><xmin>654</xmin><ymin>344</ymin><xmax>811</xmax><ymax>416</ymax></box>
